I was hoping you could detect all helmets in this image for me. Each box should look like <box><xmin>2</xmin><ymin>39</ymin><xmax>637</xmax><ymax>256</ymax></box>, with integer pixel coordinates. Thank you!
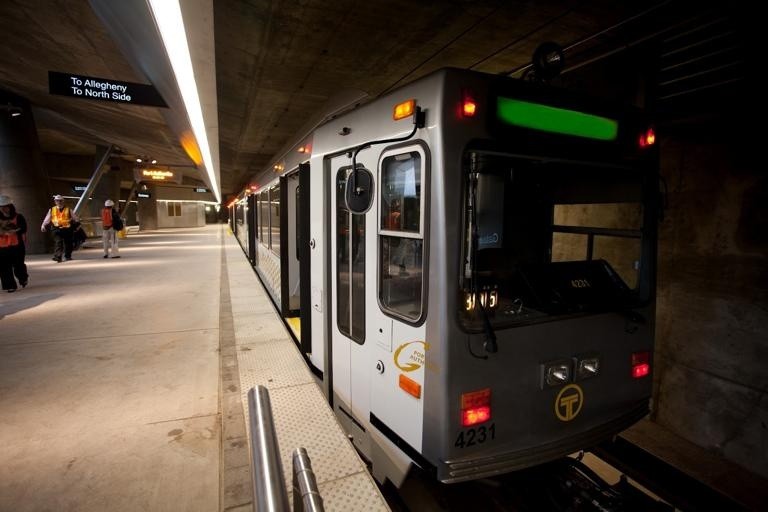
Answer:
<box><xmin>0</xmin><ymin>195</ymin><xmax>15</xmax><ymax>207</ymax></box>
<box><xmin>54</xmin><ymin>194</ymin><xmax>65</xmax><ymax>201</ymax></box>
<box><xmin>104</xmin><ymin>199</ymin><xmax>114</xmax><ymax>207</ymax></box>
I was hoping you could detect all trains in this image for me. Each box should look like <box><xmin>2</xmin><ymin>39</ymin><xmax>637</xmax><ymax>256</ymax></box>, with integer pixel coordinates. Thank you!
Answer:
<box><xmin>227</xmin><ymin>66</ymin><xmax>662</xmax><ymax>491</ymax></box>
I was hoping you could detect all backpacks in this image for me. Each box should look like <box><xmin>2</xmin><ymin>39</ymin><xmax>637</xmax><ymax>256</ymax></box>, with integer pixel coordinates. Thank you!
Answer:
<box><xmin>112</xmin><ymin>209</ymin><xmax>124</xmax><ymax>231</ymax></box>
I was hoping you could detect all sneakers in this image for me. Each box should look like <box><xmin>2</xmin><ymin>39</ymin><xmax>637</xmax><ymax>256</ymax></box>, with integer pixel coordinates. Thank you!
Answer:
<box><xmin>7</xmin><ymin>278</ymin><xmax>28</xmax><ymax>293</ymax></box>
<box><xmin>104</xmin><ymin>255</ymin><xmax>121</xmax><ymax>258</ymax></box>
<box><xmin>52</xmin><ymin>258</ymin><xmax>73</xmax><ymax>262</ymax></box>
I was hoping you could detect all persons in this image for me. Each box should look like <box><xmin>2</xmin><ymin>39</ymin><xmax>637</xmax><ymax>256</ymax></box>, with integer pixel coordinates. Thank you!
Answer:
<box><xmin>0</xmin><ymin>196</ymin><xmax>30</xmax><ymax>293</ymax></box>
<box><xmin>40</xmin><ymin>195</ymin><xmax>86</xmax><ymax>262</ymax></box>
<box><xmin>385</xmin><ymin>199</ymin><xmax>410</xmax><ymax>280</ymax></box>
<box><xmin>101</xmin><ymin>200</ymin><xmax>123</xmax><ymax>258</ymax></box>
